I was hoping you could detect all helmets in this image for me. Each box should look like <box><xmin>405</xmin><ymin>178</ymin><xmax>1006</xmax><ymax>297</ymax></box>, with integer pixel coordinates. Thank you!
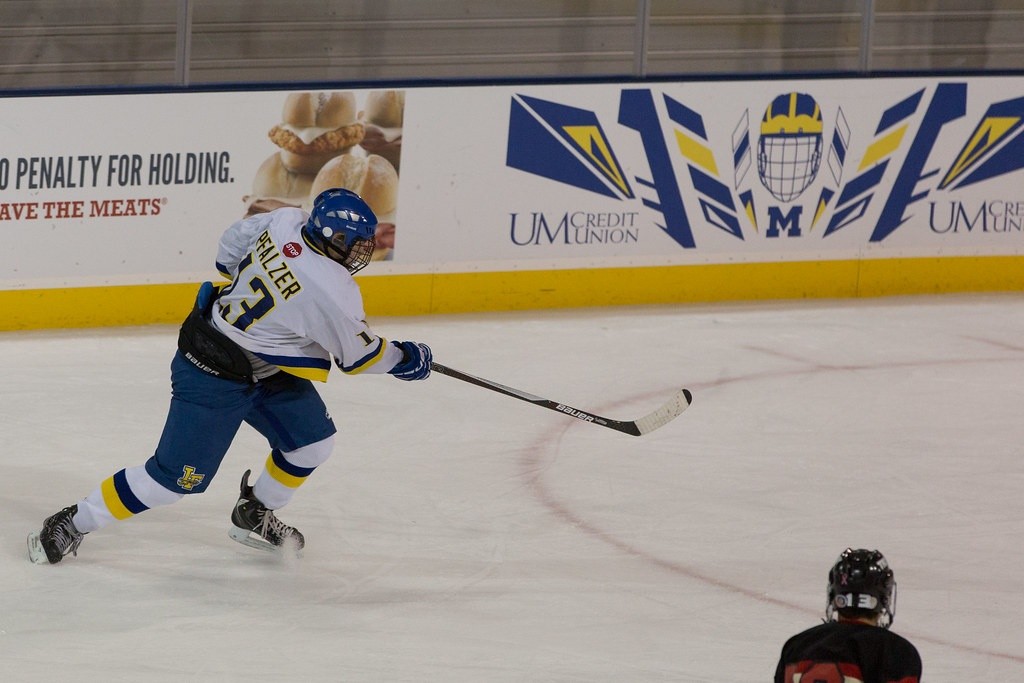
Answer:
<box><xmin>302</xmin><ymin>188</ymin><xmax>378</xmax><ymax>275</ymax></box>
<box><xmin>820</xmin><ymin>547</ymin><xmax>897</xmax><ymax>615</ymax></box>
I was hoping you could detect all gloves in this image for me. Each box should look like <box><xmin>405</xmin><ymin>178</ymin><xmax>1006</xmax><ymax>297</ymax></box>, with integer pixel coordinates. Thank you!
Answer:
<box><xmin>386</xmin><ymin>341</ymin><xmax>432</xmax><ymax>381</ymax></box>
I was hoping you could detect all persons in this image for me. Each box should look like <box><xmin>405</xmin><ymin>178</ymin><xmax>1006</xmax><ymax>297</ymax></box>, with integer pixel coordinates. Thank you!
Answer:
<box><xmin>774</xmin><ymin>547</ymin><xmax>922</xmax><ymax>683</ymax></box>
<box><xmin>26</xmin><ymin>187</ymin><xmax>433</xmax><ymax>565</ymax></box>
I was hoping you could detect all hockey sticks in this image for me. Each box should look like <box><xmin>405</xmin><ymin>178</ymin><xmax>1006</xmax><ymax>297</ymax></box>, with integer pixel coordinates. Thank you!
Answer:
<box><xmin>429</xmin><ymin>360</ymin><xmax>694</xmax><ymax>438</ymax></box>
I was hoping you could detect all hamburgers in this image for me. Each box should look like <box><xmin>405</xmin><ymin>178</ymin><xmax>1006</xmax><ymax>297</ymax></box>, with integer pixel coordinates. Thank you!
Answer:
<box><xmin>240</xmin><ymin>91</ymin><xmax>410</xmax><ymax>261</ymax></box>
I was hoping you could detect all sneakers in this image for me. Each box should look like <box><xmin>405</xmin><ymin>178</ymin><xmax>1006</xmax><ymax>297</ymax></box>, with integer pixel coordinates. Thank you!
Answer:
<box><xmin>227</xmin><ymin>469</ymin><xmax>306</xmax><ymax>560</ymax></box>
<box><xmin>27</xmin><ymin>504</ymin><xmax>89</xmax><ymax>565</ymax></box>
